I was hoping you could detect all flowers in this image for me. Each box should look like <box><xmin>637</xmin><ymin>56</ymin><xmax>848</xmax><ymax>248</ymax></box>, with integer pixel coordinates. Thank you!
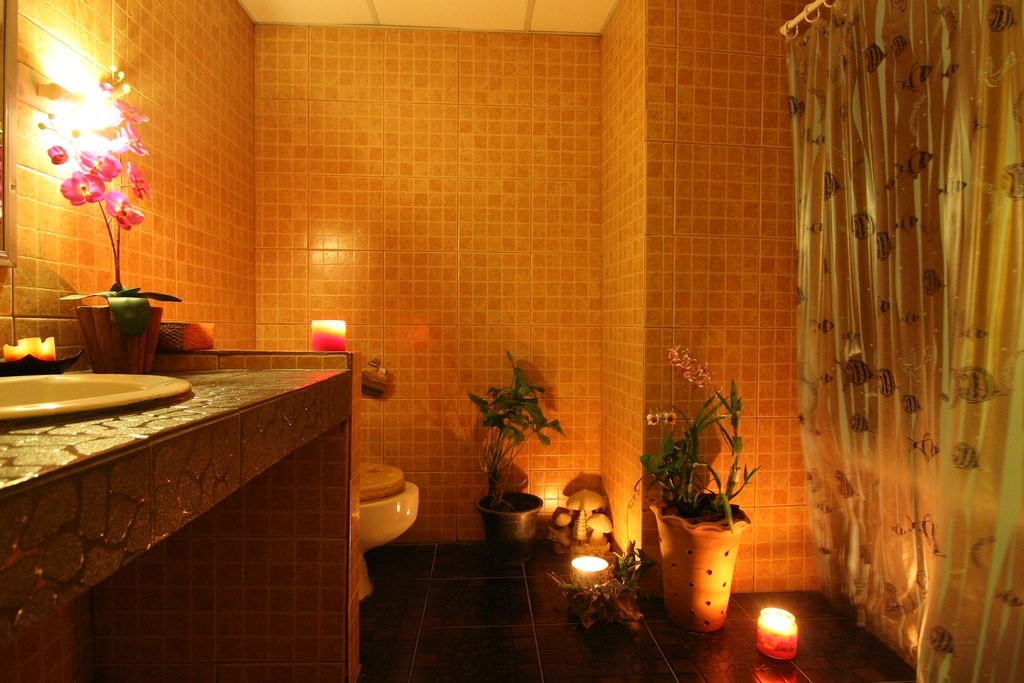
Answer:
<box><xmin>50</xmin><ymin>83</ymin><xmax>184</xmax><ymax>348</ymax></box>
<box><xmin>631</xmin><ymin>343</ymin><xmax>764</xmax><ymax>537</ymax></box>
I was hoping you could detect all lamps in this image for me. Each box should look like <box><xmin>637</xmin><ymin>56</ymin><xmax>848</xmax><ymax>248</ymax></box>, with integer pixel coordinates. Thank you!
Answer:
<box><xmin>38</xmin><ymin>84</ymin><xmax>82</xmax><ymax>104</ymax></box>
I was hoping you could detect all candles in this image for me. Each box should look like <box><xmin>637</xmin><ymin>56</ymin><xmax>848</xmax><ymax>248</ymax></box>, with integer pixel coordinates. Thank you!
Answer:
<box><xmin>756</xmin><ymin>607</ymin><xmax>798</xmax><ymax>660</ymax></box>
<box><xmin>311</xmin><ymin>320</ymin><xmax>346</xmax><ymax>351</ymax></box>
<box><xmin>571</xmin><ymin>556</ymin><xmax>609</xmax><ymax>585</ymax></box>
<box><xmin>3</xmin><ymin>336</ymin><xmax>57</xmax><ymax>361</ymax></box>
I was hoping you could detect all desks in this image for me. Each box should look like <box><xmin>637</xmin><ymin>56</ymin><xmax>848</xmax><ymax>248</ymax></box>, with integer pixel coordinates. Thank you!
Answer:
<box><xmin>0</xmin><ymin>350</ymin><xmax>364</xmax><ymax>683</ymax></box>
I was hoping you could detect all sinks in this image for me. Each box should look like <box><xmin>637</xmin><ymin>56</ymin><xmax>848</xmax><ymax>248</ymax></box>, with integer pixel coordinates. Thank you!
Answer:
<box><xmin>0</xmin><ymin>373</ymin><xmax>195</xmax><ymax>422</ymax></box>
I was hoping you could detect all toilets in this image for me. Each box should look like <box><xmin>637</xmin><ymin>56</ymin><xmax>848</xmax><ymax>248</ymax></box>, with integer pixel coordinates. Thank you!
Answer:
<box><xmin>359</xmin><ymin>461</ymin><xmax>420</xmax><ymax>599</ymax></box>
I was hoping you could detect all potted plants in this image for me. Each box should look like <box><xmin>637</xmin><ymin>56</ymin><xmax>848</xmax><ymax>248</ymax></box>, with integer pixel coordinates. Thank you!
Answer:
<box><xmin>467</xmin><ymin>349</ymin><xmax>566</xmax><ymax>567</ymax></box>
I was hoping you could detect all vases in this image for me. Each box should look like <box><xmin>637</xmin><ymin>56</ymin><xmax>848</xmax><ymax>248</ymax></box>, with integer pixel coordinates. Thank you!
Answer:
<box><xmin>74</xmin><ymin>305</ymin><xmax>165</xmax><ymax>373</ymax></box>
<box><xmin>649</xmin><ymin>496</ymin><xmax>753</xmax><ymax>633</ymax></box>
